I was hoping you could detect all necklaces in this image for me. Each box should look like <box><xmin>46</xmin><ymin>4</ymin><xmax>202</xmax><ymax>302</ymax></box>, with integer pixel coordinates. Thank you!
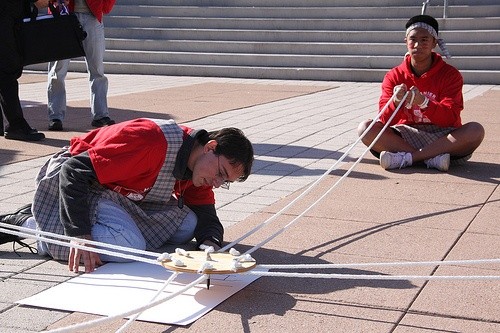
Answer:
<box><xmin>178</xmin><ymin>179</ymin><xmax>188</xmax><ymax>209</ymax></box>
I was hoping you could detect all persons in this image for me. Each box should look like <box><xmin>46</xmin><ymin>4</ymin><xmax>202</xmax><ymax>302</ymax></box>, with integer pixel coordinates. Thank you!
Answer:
<box><xmin>357</xmin><ymin>15</ymin><xmax>485</xmax><ymax>170</ymax></box>
<box><xmin>0</xmin><ymin>0</ymin><xmax>54</xmax><ymax>140</ymax></box>
<box><xmin>0</xmin><ymin>118</ymin><xmax>253</xmax><ymax>272</ymax></box>
<box><xmin>48</xmin><ymin>0</ymin><xmax>116</xmax><ymax>130</ymax></box>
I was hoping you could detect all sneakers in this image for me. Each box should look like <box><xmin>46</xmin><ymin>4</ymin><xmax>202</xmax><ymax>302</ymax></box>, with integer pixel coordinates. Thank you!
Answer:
<box><xmin>6</xmin><ymin>128</ymin><xmax>45</xmax><ymax>141</ymax></box>
<box><xmin>92</xmin><ymin>117</ymin><xmax>115</xmax><ymax>126</ymax></box>
<box><xmin>0</xmin><ymin>203</ymin><xmax>38</xmax><ymax>257</ymax></box>
<box><xmin>424</xmin><ymin>153</ymin><xmax>451</xmax><ymax>171</ymax></box>
<box><xmin>379</xmin><ymin>150</ymin><xmax>413</xmax><ymax>170</ymax></box>
<box><xmin>49</xmin><ymin>119</ymin><xmax>62</xmax><ymax>130</ymax></box>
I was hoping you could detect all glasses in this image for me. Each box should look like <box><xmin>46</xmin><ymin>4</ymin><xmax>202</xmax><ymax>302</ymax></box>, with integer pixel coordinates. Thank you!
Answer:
<box><xmin>215</xmin><ymin>154</ymin><xmax>230</xmax><ymax>189</ymax></box>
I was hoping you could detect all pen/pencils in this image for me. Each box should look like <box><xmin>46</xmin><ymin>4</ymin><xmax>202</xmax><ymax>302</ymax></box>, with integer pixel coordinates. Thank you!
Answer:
<box><xmin>207</xmin><ymin>274</ymin><xmax>210</xmax><ymax>291</ymax></box>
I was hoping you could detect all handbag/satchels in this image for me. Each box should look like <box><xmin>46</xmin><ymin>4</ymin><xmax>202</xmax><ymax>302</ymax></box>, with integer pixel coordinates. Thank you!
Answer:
<box><xmin>15</xmin><ymin>3</ymin><xmax>86</xmax><ymax>66</ymax></box>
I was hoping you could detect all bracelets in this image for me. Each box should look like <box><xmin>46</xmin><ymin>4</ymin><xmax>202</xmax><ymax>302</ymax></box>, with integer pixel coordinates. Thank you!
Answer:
<box><xmin>418</xmin><ymin>96</ymin><xmax>429</xmax><ymax>108</ymax></box>
